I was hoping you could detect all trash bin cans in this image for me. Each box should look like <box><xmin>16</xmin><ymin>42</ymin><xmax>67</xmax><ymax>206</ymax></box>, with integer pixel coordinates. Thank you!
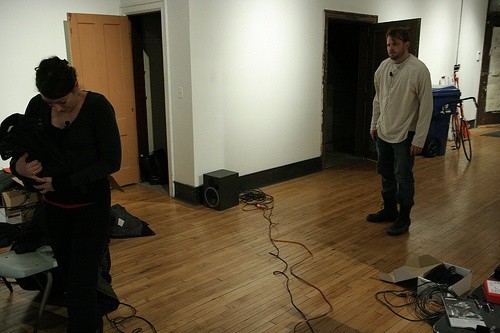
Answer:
<box><xmin>417</xmin><ymin>84</ymin><xmax>462</xmax><ymax>159</ymax></box>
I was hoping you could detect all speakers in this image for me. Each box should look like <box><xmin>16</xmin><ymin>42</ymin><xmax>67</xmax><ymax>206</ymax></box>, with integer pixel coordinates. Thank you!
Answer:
<box><xmin>203</xmin><ymin>169</ymin><xmax>239</xmax><ymax>211</ymax></box>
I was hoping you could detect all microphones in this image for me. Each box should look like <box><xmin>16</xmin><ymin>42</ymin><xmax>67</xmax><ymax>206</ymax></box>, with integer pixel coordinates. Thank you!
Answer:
<box><xmin>389</xmin><ymin>72</ymin><xmax>394</xmax><ymax>76</ymax></box>
<box><xmin>65</xmin><ymin>120</ymin><xmax>71</xmax><ymax>127</ymax></box>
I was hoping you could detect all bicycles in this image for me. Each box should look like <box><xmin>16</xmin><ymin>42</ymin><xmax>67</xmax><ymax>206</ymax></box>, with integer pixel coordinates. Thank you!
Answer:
<box><xmin>449</xmin><ymin>96</ymin><xmax>479</xmax><ymax>161</ymax></box>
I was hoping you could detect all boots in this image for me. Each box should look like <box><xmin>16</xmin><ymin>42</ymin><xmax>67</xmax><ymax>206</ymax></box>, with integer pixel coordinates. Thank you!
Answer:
<box><xmin>386</xmin><ymin>207</ymin><xmax>412</xmax><ymax>236</ymax></box>
<box><xmin>367</xmin><ymin>199</ymin><xmax>399</xmax><ymax>222</ymax></box>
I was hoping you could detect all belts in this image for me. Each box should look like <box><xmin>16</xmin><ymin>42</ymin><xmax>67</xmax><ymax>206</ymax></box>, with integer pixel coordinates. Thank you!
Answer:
<box><xmin>42</xmin><ymin>194</ymin><xmax>96</xmax><ymax>209</ymax></box>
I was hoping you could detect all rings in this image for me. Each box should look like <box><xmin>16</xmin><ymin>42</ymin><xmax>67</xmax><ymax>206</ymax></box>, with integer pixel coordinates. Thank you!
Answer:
<box><xmin>31</xmin><ymin>171</ymin><xmax>36</xmax><ymax>176</ymax></box>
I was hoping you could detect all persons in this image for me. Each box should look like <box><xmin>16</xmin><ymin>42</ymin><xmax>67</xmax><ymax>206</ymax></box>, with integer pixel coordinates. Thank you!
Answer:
<box><xmin>366</xmin><ymin>27</ymin><xmax>434</xmax><ymax>236</ymax></box>
<box><xmin>10</xmin><ymin>56</ymin><xmax>121</xmax><ymax>333</ymax></box>
